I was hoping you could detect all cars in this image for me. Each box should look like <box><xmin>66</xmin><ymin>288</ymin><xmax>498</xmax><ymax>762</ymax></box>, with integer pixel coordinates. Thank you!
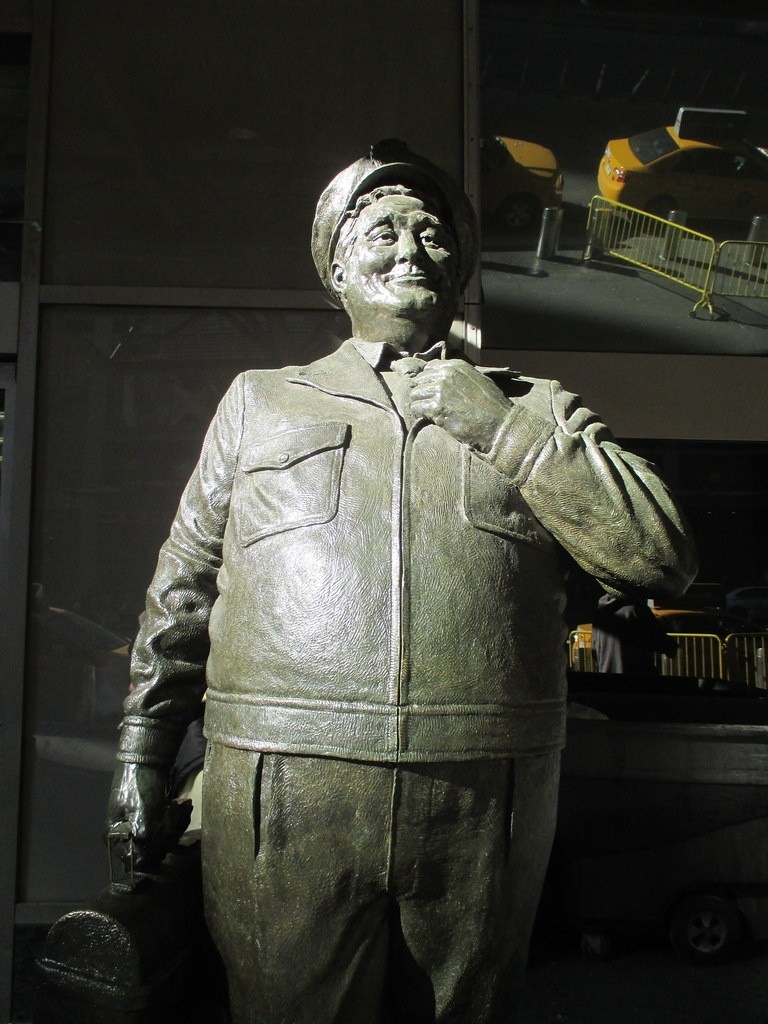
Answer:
<box><xmin>597</xmin><ymin>108</ymin><xmax>768</xmax><ymax>237</ymax></box>
<box><xmin>480</xmin><ymin>135</ymin><xmax>564</xmax><ymax>234</ymax></box>
<box><xmin>725</xmin><ymin>586</ymin><xmax>768</xmax><ymax>620</ymax></box>
<box><xmin>572</xmin><ymin>610</ymin><xmax>768</xmax><ymax>686</ymax></box>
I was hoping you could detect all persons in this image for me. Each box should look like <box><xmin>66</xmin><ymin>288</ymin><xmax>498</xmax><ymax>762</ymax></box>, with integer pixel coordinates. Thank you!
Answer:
<box><xmin>105</xmin><ymin>144</ymin><xmax>699</xmax><ymax>1024</ymax></box>
<box><xmin>591</xmin><ymin>590</ymin><xmax>680</xmax><ymax>677</ymax></box>
